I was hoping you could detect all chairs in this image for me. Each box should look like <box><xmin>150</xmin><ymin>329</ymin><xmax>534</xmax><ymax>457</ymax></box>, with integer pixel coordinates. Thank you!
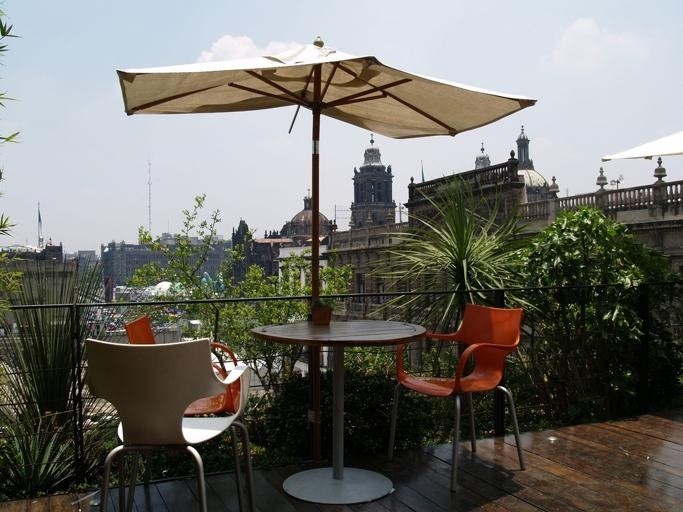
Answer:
<box><xmin>386</xmin><ymin>303</ymin><xmax>525</xmax><ymax>493</ymax></box>
<box><xmin>116</xmin><ymin>316</ymin><xmax>256</xmax><ymax>512</ymax></box>
<box><xmin>85</xmin><ymin>338</ymin><xmax>253</xmax><ymax>512</ymax></box>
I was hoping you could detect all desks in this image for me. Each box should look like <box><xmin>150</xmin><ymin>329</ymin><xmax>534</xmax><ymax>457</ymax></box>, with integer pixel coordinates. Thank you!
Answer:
<box><xmin>250</xmin><ymin>321</ymin><xmax>427</xmax><ymax>505</ymax></box>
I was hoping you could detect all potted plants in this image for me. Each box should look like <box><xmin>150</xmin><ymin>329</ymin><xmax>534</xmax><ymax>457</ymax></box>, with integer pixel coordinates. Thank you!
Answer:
<box><xmin>308</xmin><ymin>299</ymin><xmax>332</xmax><ymax>327</ymax></box>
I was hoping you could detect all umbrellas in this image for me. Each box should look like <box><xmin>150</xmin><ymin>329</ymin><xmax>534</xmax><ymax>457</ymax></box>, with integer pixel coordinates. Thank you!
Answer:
<box><xmin>116</xmin><ymin>34</ymin><xmax>539</xmax><ymax>468</ymax></box>
<box><xmin>601</xmin><ymin>129</ymin><xmax>683</xmax><ymax>161</ymax></box>
